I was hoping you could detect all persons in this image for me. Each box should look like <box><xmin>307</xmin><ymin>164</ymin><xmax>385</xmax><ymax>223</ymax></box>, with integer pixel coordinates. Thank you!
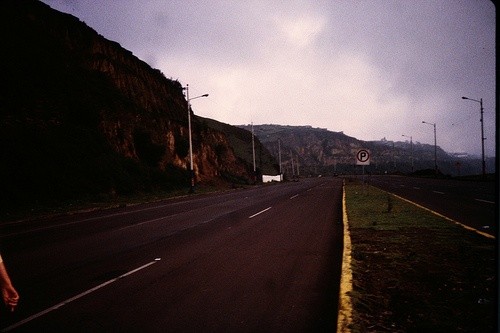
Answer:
<box><xmin>0</xmin><ymin>255</ymin><xmax>19</xmax><ymax>311</ymax></box>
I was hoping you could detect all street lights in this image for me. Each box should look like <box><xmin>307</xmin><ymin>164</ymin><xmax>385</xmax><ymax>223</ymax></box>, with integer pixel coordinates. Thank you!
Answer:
<box><xmin>277</xmin><ymin>137</ymin><xmax>299</xmax><ymax>178</ymax></box>
<box><xmin>185</xmin><ymin>85</ymin><xmax>208</xmax><ymax>193</ymax></box>
<box><xmin>401</xmin><ymin>135</ymin><xmax>413</xmax><ymax>170</ymax></box>
<box><xmin>421</xmin><ymin>120</ymin><xmax>438</xmax><ymax>168</ymax></box>
<box><xmin>461</xmin><ymin>96</ymin><xmax>487</xmax><ymax>177</ymax></box>
<box><xmin>251</xmin><ymin>122</ymin><xmax>265</xmax><ymax>181</ymax></box>
